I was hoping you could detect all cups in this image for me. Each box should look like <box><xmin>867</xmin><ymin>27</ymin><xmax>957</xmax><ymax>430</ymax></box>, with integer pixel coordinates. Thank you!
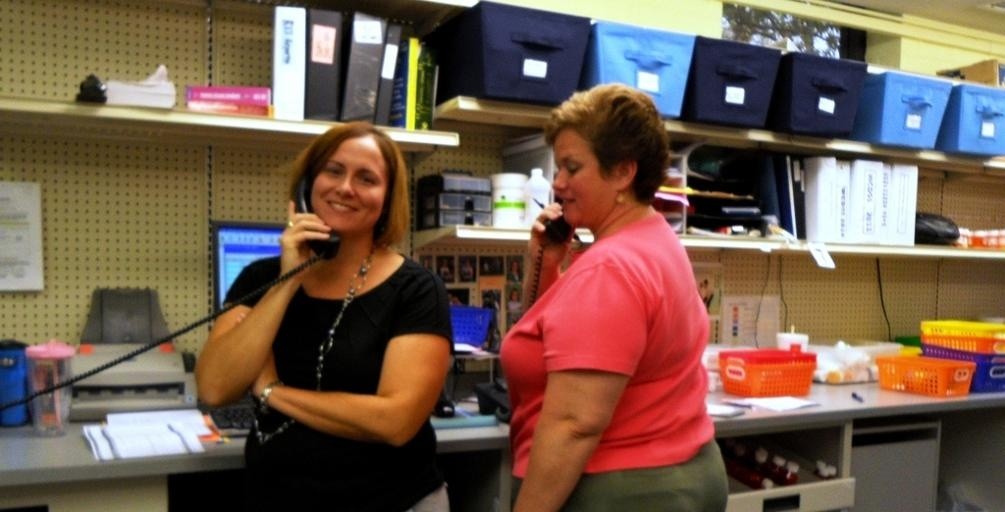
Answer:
<box><xmin>775</xmin><ymin>333</ymin><xmax>809</xmax><ymax>351</ymax></box>
<box><xmin>24</xmin><ymin>343</ymin><xmax>76</xmax><ymax>437</ymax></box>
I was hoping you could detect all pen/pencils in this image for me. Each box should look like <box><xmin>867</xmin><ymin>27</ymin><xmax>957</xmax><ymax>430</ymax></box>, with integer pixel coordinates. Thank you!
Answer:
<box><xmin>851</xmin><ymin>391</ymin><xmax>865</xmax><ymax>404</ymax></box>
<box><xmin>723</xmin><ymin>399</ymin><xmax>755</xmax><ymax>411</ymax></box>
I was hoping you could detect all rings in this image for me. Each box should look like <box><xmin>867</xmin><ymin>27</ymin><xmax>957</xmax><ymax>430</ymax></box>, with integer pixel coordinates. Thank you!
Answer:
<box><xmin>283</xmin><ymin>219</ymin><xmax>295</xmax><ymax>229</ymax></box>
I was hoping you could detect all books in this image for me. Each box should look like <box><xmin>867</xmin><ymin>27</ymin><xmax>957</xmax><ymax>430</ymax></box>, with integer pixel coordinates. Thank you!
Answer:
<box><xmin>398</xmin><ymin>36</ymin><xmax>440</xmax><ymax>134</ymax></box>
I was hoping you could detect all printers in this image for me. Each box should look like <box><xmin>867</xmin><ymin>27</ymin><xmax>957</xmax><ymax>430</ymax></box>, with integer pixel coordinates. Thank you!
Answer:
<box><xmin>61</xmin><ymin>287</ymin><xmax>197</xmax><ymax>421</ymax></box>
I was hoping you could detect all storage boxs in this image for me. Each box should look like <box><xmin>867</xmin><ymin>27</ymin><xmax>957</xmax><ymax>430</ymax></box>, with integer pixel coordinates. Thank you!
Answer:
<box><xmin>847</xmin><ymin>72</ymin><xmax>953</xmax><ymax>150</ymax></box>
<box><xmin>582</xmin><ymin>20</ymin><xmax>695</xmax><ymax>124</ymax></box>
<box><xmin>921</xmin><ymin>344</ymin><xmax>1004</xmax><ymax>392</ymax></box>
<box><xmin>451</xmin><ymin>303</ymin><xmax>494</xmax><ymax>345</ymax></box>
<box><xmin>857</xmin><ymin>155</ymin><xmax>889</xmax><ymax>247</ymax></box>
<box><xmin>419</xmin><ymin>1</ymin><xmax>592</xmax><ymax>107</ymax></box>
<box><xmin>764</xmin><ymin>52</ymin><xmax>868</xmax><ymax>138</ymax></box>
<box><xmin>814</xmin><ymin>336</ymin><xmax>904</xmax><ymax>385</ymax></box>
<box><xmin>920</xmin><ymin>318</ymin><xmax>1004</xmax><ymax>351</ymax></box>
<box><xmin>682</xmin><ymin>36</ymin><xmax>783</xmax><ymax>129</ymax></box>
<box><xmin>806</xmin><ymin>154</ymin><xmax>852</xmax><ymax>245</ymax></box>
<box><xmin>889</xmin><ymin>160</ymin><xmax>918</xmax><ymax>249</ymax></box>
<box><xmin>719</xmin><ymin>348</ymin><xmax>817</xmax><ymax>398</ymax></box>
<box><xmin>935</xmin><ymin>84</ymin><xmax>1005</xmax><ymax>156</ymax></box>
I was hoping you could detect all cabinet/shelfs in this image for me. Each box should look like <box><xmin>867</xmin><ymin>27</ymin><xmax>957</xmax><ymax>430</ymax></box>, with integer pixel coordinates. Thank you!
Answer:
<box><xmin>851</xmin><ymin>423</ymin><xmax>943</xmax><ymax>511</ymax></box>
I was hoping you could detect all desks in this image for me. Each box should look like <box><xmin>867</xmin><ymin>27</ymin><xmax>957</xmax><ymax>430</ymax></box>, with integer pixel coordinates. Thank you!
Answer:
<box><xmin>706</xmin><ymin>374</ymin><xmax>1005</xmax><ymax>512</ymax></box>
<box><xmin>0</xmin><ymin>413</ymin><xmax>517</xmax><ymax>512</ymax></box>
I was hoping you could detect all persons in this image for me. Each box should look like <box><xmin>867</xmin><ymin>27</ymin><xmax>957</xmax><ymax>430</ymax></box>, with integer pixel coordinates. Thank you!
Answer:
<box><xmin>194</xmin><ymin>122</ymin><xmax>452</xmax><ymax>510</ymax></box>
<box><xmin>500</xmin><ymin>83</ymin><xmax>729</xmax><ymax>512</ymax></box>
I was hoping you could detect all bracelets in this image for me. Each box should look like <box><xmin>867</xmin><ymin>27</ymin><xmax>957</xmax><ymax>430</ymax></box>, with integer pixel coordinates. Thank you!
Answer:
<box><xmin>259</xmin><ymin>380</ymin><xmax>286</xmax><ymax>413</ymax></box>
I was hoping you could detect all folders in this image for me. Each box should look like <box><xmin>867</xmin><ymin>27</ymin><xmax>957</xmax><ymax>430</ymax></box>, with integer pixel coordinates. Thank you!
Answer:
<box><xmin>343</xmin><ymin>4</ymin><xmax>389</xmax><ymax>124</ymax></box>
<box><xmin>273</xmin><ymin>4</ymin><xmax>306</xmax><ymax>123</ymax></box>
<box><xmin>376</xmin><ymin>22</ymin><xmax>405</xmax><ymax>126</ymax></box>
<box><xmin>307</xmin><ymin>7</ymin><xmax>343</xmax><ymax>124</ymax></box>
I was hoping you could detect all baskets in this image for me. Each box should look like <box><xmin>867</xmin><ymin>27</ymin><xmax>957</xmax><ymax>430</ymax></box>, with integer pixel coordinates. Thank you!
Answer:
<box><xmin>720</xmin><ymin>344</ymin><xmax>817</xmax><ymax>363</ymax></box>
<box><xmin>922</xmin><ymin>320</ymin><xmax>1005</xmax><ymax>337</ymax></box>
<box><xmin>720</xmin><ymin>360</ymin><xmax>817</xmax><ymax>398</ymax></box>
<box><xmin>923</xmin><ymin>343</ymin><xmax>1004</xmax><ymax>363</ymax></box>
<box><xmin>922</xmin><ymin>354</ymin><xmax>1005</xmax><ymax>393</ymax></box>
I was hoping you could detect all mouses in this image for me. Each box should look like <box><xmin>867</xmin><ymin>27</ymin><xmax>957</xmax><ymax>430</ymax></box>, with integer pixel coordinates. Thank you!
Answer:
<box><xmin>433</xmin><ymin>395</ymin><xmax>455</xmax><ymax>419</ymax></box>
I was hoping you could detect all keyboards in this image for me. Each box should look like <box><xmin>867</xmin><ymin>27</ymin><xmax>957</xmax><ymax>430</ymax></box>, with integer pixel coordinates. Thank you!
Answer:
<box><xmin>210</xmin><ymin>396</ymin><xmax>253</xmax><ymax>437</ymax></box>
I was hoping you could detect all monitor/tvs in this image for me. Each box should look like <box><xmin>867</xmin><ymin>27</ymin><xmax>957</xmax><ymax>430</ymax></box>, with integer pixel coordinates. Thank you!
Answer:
<box><xmin>212</xmin><ymin>220</ymin><xmax>286</xmax><ymax>325</ymax></box>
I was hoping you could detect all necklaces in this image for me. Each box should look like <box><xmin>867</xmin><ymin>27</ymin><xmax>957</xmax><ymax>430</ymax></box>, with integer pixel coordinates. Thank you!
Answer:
<box><xmin>252</xmin><ymin>247</ymin><xmax>371</xmax><ymax>446</ymax></box>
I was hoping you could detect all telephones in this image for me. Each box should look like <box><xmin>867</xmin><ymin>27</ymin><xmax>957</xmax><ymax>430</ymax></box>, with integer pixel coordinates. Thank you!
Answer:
<box><xmin>540</xmin><ymin>193</ymin><xmax>574</xmax><ymax>248</ymax></box>
<box><xmin>472</xmin><ymin>378</ymin><xmax>513</xmax><ymax>424</ymax></box>
<box><xmin>291</xmin><ymin>170</ymin><xmax>344</xmax><ymax>262</ymax></box>
